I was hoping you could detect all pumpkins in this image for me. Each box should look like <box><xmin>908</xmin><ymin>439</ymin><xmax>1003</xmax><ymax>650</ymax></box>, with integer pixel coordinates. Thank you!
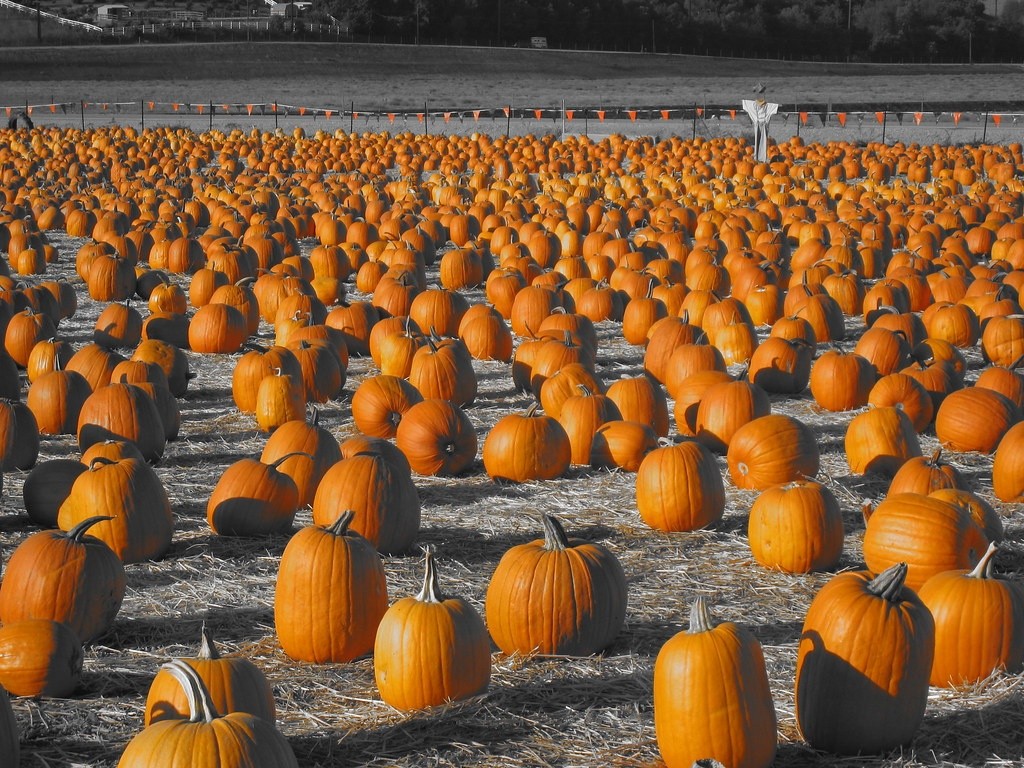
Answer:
<box><xmin>0</xmin><ymin>123</ymin><xmax>1024</xmax><ymax>768</ymax></box>
